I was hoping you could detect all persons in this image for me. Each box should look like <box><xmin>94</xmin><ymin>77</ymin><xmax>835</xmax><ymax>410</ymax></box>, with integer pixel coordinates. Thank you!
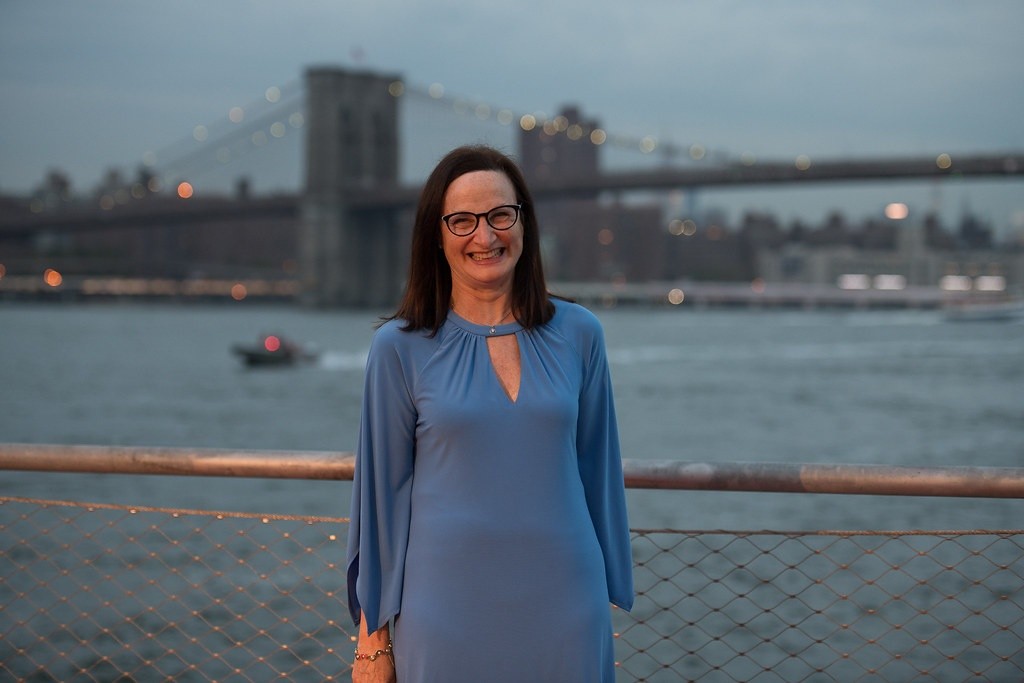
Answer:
<box><xmin>347</xmin><ymin>147</ymin><xmax>633</xmax><ymax>683</ymax></box>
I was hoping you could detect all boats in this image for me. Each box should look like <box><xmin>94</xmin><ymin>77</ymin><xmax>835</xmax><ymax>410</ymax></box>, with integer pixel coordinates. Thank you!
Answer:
<box><xmin>227</xmin><ymin>331</ymin><xmax>321</xmax><ymax>365</ymax></box>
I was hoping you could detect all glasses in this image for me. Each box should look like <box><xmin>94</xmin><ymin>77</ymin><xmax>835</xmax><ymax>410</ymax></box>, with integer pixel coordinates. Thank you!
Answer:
<box><xmin>440</xmin><ymin>205</ymin><xmax>522</xmax><ymax>237</ymax></box>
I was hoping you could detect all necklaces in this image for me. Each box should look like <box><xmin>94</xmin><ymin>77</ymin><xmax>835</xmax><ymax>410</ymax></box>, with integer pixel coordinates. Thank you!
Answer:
<box><xmin>452</xmin><ymin>304</ymin><xmax>511</xmax><ymax>335</ymax></box>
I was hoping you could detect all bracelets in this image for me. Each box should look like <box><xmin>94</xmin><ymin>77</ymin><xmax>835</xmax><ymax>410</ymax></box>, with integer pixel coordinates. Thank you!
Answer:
<box><xmin>354</xmin><ymin>638</ymin><xmax>392</xmax><ymax>660</ymax></box>
